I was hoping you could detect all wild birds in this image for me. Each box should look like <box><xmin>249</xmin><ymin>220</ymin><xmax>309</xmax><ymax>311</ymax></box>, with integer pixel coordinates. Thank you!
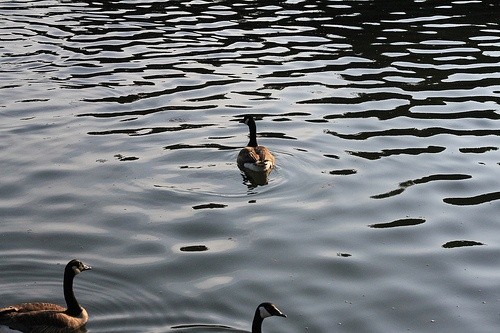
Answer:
<box><xmin>170</xmin><ymin>302</ymin><xmax>289</xmax><ymax>333</ymax></box>
<box><xmin>0</xmin><ymin>259</ymin><xmax>94</xmax><ymax>333</ymax></box>
<box><xmin>236</xmin><ymin>114</ymin><xmax>275</xmax><ymax>174</ymax></box>
